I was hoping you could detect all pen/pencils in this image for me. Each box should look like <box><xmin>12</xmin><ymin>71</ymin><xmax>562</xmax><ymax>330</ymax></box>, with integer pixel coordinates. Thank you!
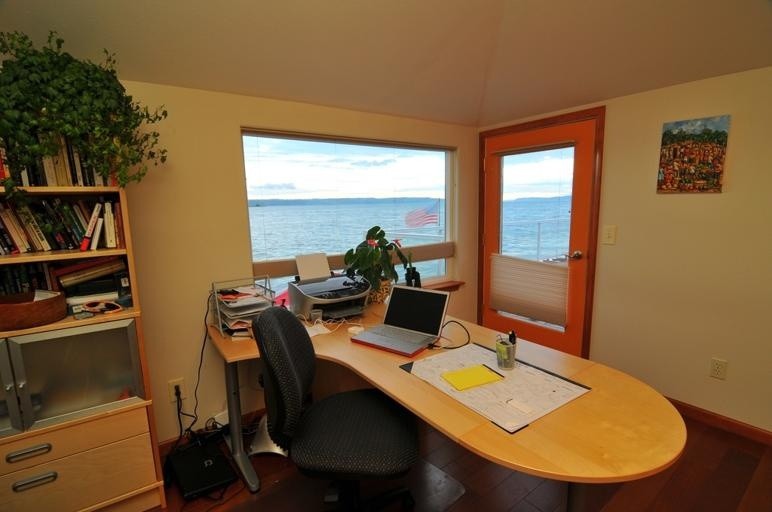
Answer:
<box><xmin>509</xmin><ymin>330</ymin><xmax>516</xmax><ymax>344</ymax></box>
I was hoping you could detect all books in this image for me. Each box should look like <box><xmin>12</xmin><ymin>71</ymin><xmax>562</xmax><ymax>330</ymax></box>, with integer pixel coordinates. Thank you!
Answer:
<box><xmin>0</xmin><ymin>117</ymin><xmax>134</xmax><ymax>323</ymax></box>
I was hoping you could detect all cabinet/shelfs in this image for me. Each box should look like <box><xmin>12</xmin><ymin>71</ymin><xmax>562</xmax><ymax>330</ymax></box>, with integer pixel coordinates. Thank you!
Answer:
<box><xmin>2</xmin><ymin>181</ymin><xmax>167</xmax><ymax>511</ymax></box>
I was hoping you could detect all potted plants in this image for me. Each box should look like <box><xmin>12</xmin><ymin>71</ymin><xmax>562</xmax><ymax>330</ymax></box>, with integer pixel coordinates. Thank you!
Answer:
<box><xmin>344</xmin><ymin>226</ymin><xmax>414</xmax><ymax>305</ymax></box>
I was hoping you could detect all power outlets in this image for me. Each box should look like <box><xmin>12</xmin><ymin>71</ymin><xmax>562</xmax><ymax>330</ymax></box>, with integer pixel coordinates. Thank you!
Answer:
<box><xmin>708</xmin><ymin>357</ymin><xmax>728</xmax><ymax>380</ymax></box>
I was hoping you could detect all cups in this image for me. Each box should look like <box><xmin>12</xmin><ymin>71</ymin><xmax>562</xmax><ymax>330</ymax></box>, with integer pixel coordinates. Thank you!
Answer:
<box><xmin>311</xmin><ymin>309</ymin><xmax>323</xmax><ymax>324</ymax></box>
<box><xmin>497</xmin><ymin>340</ymin><xmax>517</xmax><ymax>370</ymax></box>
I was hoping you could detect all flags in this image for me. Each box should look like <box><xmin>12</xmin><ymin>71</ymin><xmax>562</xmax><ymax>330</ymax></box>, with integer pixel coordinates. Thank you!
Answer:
<box><xmin>405</xmin><ymin>200</ymin><xmax>439</xmax><ymax>228</ymax></box>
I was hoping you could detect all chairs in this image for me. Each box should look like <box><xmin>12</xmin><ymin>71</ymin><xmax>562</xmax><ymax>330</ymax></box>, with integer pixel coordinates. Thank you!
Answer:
<box><xmin>252</xmin><ymin>305</ymin><xmax>410</xmax><ymax>511</ymax></box>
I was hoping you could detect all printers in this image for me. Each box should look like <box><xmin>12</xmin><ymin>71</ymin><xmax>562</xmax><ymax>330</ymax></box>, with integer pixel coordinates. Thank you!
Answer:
<box><xmin>287</xmin><ymin>252</ymin><xmax>371</xmax><ymax>323</ymax></box>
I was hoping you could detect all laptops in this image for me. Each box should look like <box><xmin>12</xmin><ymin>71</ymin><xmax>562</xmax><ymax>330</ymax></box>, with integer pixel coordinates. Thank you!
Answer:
<box><xmin>351</xmin><ymin>284</ymin><xmax>450</xmax><ymax>358</ymax></box>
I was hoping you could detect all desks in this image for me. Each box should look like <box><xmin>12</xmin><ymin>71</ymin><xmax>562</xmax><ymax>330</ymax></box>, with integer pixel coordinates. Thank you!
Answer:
<box><xmin>206</xmin><ymin>298</ymin><xmax>688</xmax><ymax>510</ymax></box>
